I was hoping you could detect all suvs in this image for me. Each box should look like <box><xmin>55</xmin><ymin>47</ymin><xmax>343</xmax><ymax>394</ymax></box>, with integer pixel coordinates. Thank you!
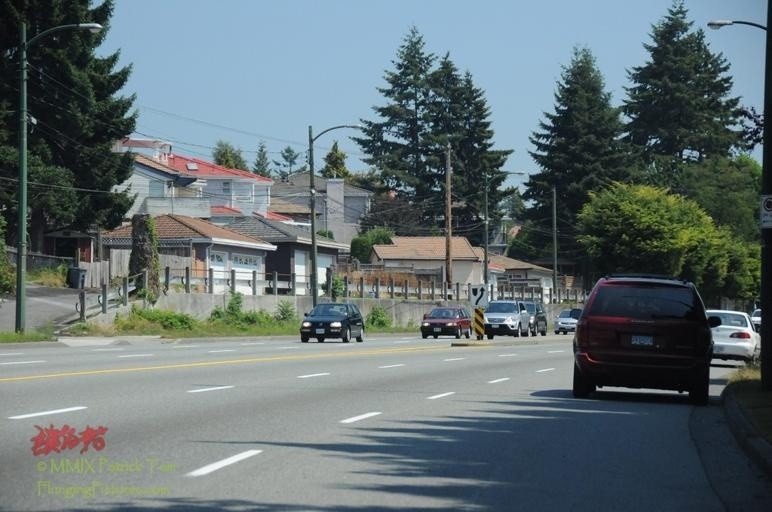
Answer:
<box><xmin>751</xmin><ymin>309</ymin><xmax>761</xmax><ymax>334</ymax></box>
<box><xmin>572</xmin><ymin>272</ymin><xmax>722</xmax><ymax>406</ymax></box>
<box><xmin>483</xmin><ymin>299</ymin><xmax>532</xmax><ymax>338</ymax></box>
<box><xmin>526</xmin><ymin>301</ymin><xmax>549</xmax><ymax>336</ymax></box>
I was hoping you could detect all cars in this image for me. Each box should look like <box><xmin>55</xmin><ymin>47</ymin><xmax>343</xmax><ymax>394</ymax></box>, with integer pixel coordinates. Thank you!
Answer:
<box><xmin>419</xmin><ymin>306</ymin><xmax>475</xmax><ymax>340</ymax></box>
<box><xmin>703</xmin><ymin>308</ymin><xmax>761</xmax><ymax>364</ymax></box>
<box><xmin>554</xmin><ymin>309</ymin><xmax>578</xmax><ymax>336</ymax></box>
<box><xmin>299</xmin><ymin>302</ymin><xmax>367</xmax><ymax>343</ymax></box>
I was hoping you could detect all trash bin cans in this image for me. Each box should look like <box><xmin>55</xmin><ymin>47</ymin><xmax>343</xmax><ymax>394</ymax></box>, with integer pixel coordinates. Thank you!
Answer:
<box><xmin>65</xmin><ymin>267</ymin><xmax>87</xmax><ymax>289</ymax></box>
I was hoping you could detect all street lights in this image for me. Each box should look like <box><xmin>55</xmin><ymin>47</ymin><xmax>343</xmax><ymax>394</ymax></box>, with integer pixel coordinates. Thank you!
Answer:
<box><xmin>14</xmin><ymin>21</ymin><xmax>105</xmax><ymax>335</ymax></box>
<box><xmin>305</xmin><ymin>123</ymin><xmax>363</xmax><ymax>307</ymax></box>
<box><xmin>705</xmin><ymin>19</ymin><xmax>771</xmax><ymax>443</ymax></box>
<box><xmin>481</xmin><ymin>169</ymin><xmax>526</xmax><ymax>285</ymax></box>
